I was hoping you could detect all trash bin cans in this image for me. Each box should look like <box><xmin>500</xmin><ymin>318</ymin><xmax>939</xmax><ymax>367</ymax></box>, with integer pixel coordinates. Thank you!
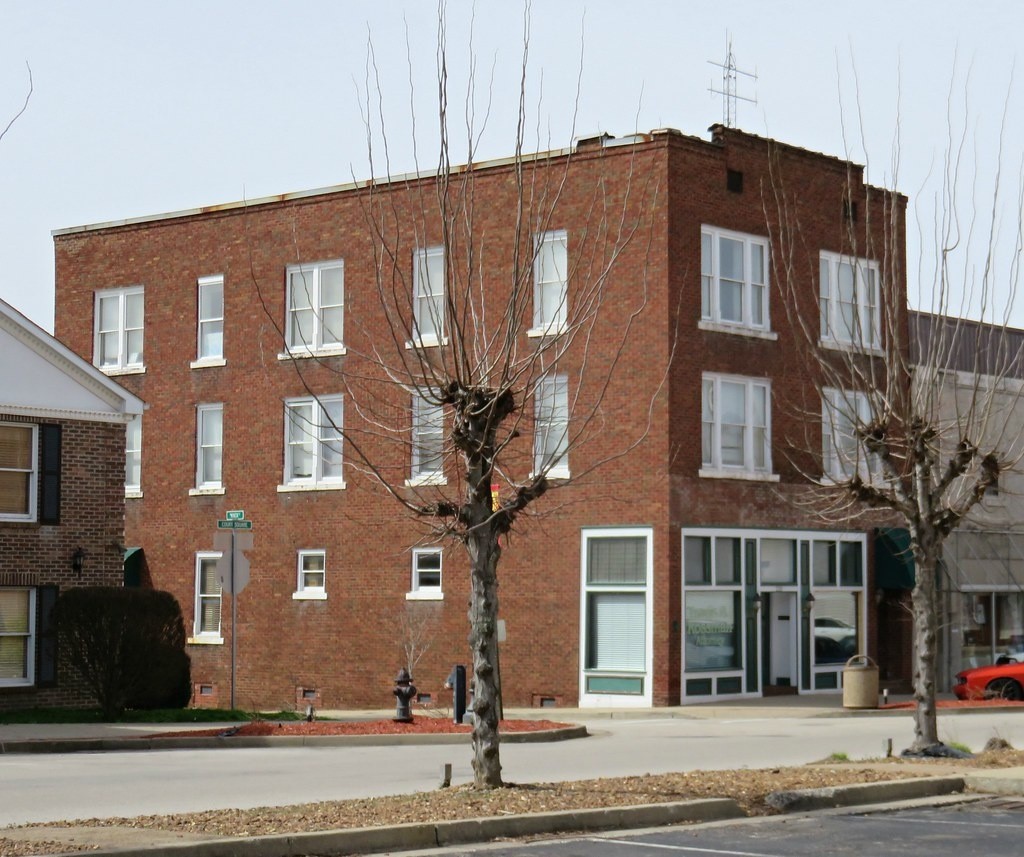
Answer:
<box><xmin>842</xmin><ymin>655</ymin><xmax>880</xmax><ymax>709</ymax></box>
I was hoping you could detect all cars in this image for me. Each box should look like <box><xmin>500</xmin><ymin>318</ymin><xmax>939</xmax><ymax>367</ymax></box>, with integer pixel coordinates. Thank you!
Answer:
<box><xmin>952</xmin><ymin>661</ymin><xmax>1024</xmax><ymax>701</ymax></box>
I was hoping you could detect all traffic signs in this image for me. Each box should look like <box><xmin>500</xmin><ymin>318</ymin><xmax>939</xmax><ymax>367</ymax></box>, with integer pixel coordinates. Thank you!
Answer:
<box><xmin>226</xmin><ymin>511</ymin><xmax>245</xmax><ymax>519</ymax></box>
<box><xmin>217</xmin><ymin>519</ymin><xmax>252</xmax><ymax>529</ymax></box>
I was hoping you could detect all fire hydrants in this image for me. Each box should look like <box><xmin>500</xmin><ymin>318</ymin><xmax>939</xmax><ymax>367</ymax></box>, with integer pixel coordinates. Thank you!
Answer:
<box><xmin>393</xmin><ymin>666</ymin><xmax>417</xmax><ymax>722</ymax></box>
